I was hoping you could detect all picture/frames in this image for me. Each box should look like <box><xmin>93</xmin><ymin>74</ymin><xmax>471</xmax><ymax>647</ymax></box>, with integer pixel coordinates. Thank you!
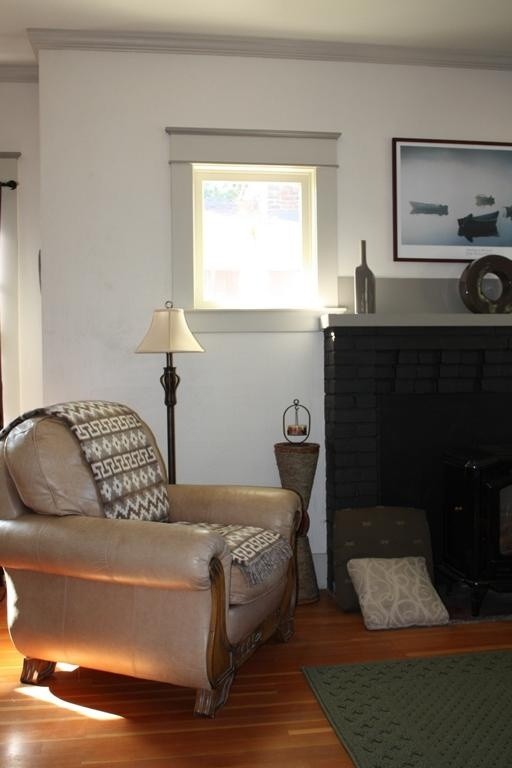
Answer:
<box><xmin>392</xmin><ymin>138</ymin><xmax>512</xmax><ymax>264</ymax></box>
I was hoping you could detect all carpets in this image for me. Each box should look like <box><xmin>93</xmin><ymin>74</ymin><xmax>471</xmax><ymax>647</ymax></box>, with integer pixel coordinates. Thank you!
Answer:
<box><xmin>299</xmin><ymin>649</ymin><xmax>512</xmax><ymax>767</ymax></box>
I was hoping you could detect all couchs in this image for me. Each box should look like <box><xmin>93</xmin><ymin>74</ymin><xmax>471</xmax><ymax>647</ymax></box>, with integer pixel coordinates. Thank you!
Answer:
<box><xmin>0</xmin><ymin>400</ymin><xmax>315</xmax><ymax>715</ymax></box>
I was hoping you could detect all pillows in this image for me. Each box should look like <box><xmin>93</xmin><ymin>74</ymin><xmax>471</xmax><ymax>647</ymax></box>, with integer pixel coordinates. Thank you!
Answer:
<box><xmin>335</xmin><ymin>500</ymin><xmax>451</xmax><ymax>632</ymax></box>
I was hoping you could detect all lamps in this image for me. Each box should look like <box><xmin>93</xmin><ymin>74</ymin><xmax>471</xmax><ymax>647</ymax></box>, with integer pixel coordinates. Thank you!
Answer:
<box><xmin>133</xmin><ymin>300</ymin><xmax>205</xmax><ymax>485</ymax></box>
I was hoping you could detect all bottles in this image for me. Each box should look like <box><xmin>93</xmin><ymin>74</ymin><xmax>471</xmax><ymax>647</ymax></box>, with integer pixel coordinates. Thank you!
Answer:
<box><xmin>356</xmin><ymin>240</ymin><xmax>375</xmax><ymax>312</ymax></box>
